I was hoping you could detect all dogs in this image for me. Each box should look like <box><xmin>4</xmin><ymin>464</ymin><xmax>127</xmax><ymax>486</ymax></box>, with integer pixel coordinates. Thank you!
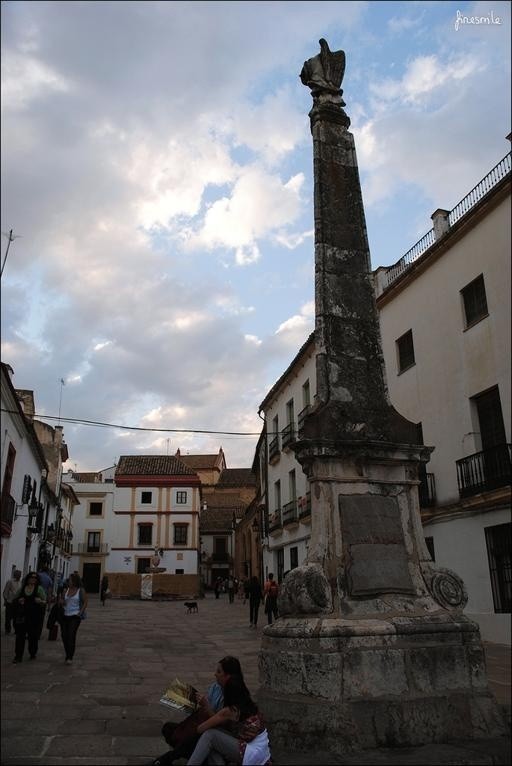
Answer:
<box><xmin>183</xmin><ymin>601</ymin><xmax>199</xmax><ymax>613</ymax></box>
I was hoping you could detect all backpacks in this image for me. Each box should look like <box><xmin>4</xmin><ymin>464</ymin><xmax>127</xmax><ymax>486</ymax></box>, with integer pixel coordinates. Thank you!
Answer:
<box><xmin>267</xmin><ymin>582</ymin><xmax>280</xmax><ymax>602</ymax></box>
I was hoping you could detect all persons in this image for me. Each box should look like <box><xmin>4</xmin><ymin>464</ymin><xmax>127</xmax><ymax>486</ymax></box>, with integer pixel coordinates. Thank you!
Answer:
<box><xmin>3</xmin><ymin>567</ymin><xmax>89</xmax><ymax>665</ymax></box>
<box><xmin>101</xmin><ymin>576</ymin><xmax>108</xmax><ymax>606</ymax></box>
<box><xmin>152</xmin><ymin>656</ymin><xmax>272</xmax><ymax>766</ymax></box>
<box><xmin>214</xmin><ymin>573</ymin><xmax>280</xmax><ymax>628</ymax></box>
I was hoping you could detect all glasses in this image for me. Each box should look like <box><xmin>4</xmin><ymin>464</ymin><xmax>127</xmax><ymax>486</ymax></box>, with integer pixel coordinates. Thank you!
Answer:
<box><xmin>31</xmin><ymin>576</ymin><xmax>37</xmax><ymax>579</ymax></box>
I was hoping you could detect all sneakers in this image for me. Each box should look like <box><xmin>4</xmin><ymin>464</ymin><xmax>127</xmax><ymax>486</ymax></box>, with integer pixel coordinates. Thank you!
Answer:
<box><xmin>249</xmin><ymin>622</ymin><xmax>257</xmax><ymax>629</ymax></box>
<box><xmin>28</xmin><ymin>651</ymin><xmax>36</xmax><ymax>660</ymax></box>
<box><xmin>12</xmin><ymin>654</ymin><xmax>23</xmax><ymax>663</ymax></box>
<box><xmin>64</xmin><ymin>653</ymin><xmax>73</xmax><ymax>665</ymax></box>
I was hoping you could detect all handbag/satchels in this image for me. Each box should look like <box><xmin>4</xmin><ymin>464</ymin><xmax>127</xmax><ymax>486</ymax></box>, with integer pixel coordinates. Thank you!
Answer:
<box><xmin>79</xmin><ymin>604</ymin><xmax>87</xmax><ymax>620</ymax></box>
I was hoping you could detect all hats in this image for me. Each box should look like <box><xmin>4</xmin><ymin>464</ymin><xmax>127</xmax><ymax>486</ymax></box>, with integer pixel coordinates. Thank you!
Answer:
<box><xmin>224</xmin><ymin>655</ymin><xmax>241</xmax><ymax>677</ymax></box>
